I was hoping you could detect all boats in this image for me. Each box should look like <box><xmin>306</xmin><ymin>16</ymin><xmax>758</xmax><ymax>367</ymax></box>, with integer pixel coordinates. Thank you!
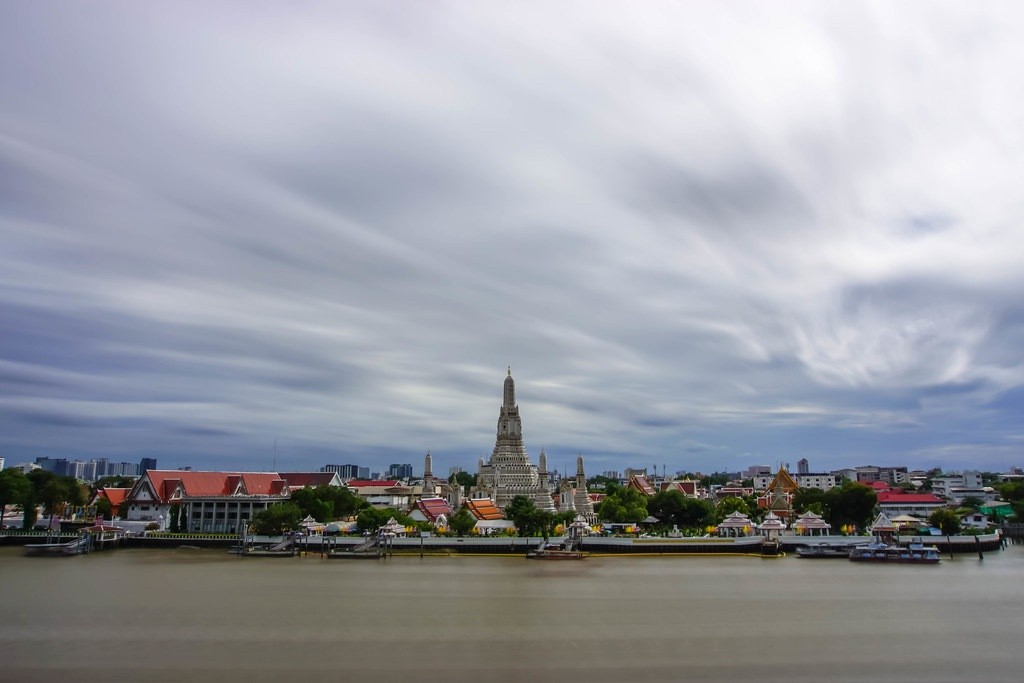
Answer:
<box><xmin>846</xmin><ymin>537</ymin><xmax>942</xmax><ymax>563</ymax></box>
<box><xmin>794</xmin><ymin>541</ymin><xmax>851</xmax><ymax>557</ymax></box>
<box><xmin>94</xmin><ymin>529</ymin><xmax>126</xmax><ymax>551</ymax></box>
